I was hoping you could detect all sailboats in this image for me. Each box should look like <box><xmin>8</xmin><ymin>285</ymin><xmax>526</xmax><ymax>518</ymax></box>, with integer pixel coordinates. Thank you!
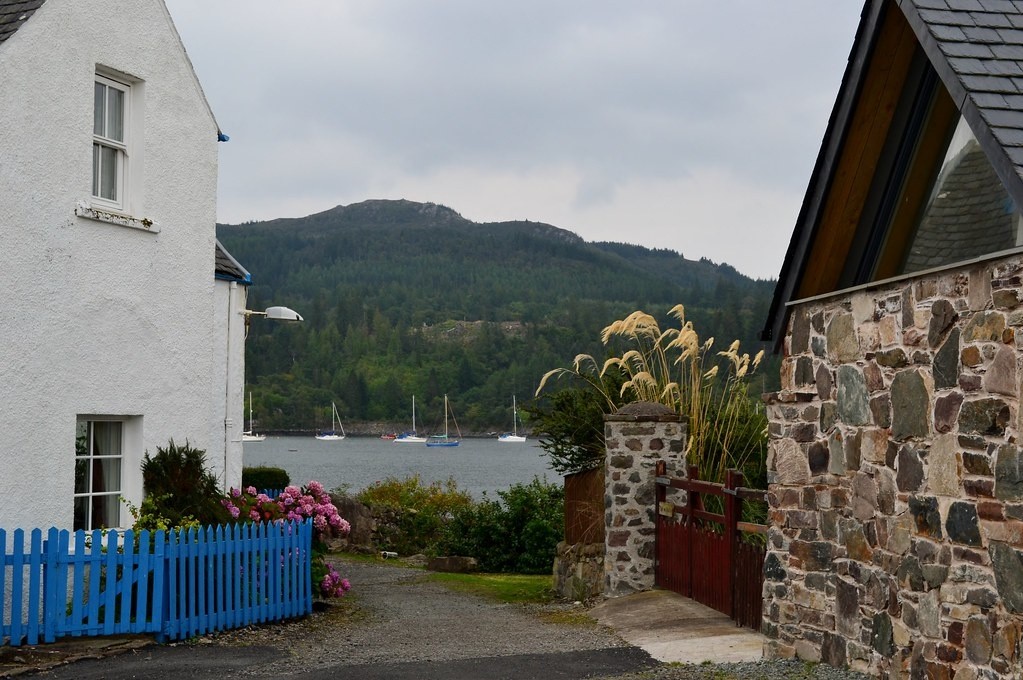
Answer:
<box><xmin>314</xmin><ymin>402</ymin><xmax>346</xmax><ymax>441</ymax></box>
<box><xmin>495</xmin><ymin>394</ymin><xmax>528</xmax><ymax>443</ymax></box>
<box><xmin>424</xmin><ymin>394</ymin><xmax>464</xmax><ymax>447</ymax></box>
<box><xmin>392</xmin><ymin>395</ymin><xmax>429</xmax><ymax>442</ymax></box>
<box><xmin>243</xmin><ymin>391</ymin><xmax>268</xmax><ymax>442</ymax></box>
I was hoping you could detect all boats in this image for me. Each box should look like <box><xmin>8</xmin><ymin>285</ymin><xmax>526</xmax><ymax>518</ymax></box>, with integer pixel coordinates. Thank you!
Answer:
<box><xmin>380</xmin><ymin>431</ymin><xmax>402</xmax><ymax>440</ymax></box>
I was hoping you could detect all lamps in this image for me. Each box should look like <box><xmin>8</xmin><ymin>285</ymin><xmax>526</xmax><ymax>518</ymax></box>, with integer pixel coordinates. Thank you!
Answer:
<box><xmin>239</xmin><ymin>306</ymin><xmax>304</xmax><ymax>321</ymax></box>
